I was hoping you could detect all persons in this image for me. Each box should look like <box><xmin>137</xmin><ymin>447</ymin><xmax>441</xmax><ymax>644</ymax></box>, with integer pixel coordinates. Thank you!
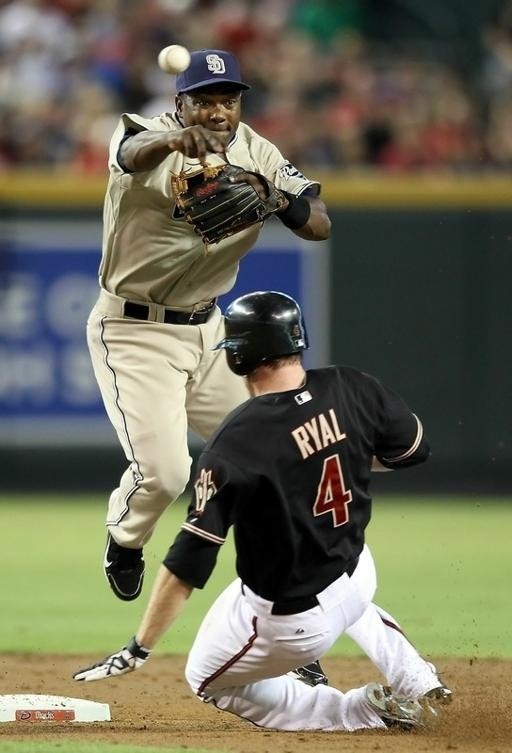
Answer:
<box><xmin>84</xmin><ymin>49</ymin><xmax>333</xmax><ymax>600</ymax></box>
<box><xmin>71</xmin><ymin>289</ymin><xmax>453</xmax><ymax>737</ymax></box>
<box><xmin>2</xmin><ymin>1</ymin><xmax>512</xmax><ymax>169</ymax></box>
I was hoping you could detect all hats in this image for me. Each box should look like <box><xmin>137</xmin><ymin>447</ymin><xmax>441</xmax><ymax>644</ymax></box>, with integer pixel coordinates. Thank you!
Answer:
<box><xmin>175</xmin><ymin>49</ymin><xmax>251</xmax><ymax>96</ymax></box>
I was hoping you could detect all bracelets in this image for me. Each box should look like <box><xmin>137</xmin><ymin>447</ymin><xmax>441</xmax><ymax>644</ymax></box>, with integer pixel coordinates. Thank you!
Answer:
<box><xmin>271</xmin><ymin>184</ymin><xmax>309</xmax><ymax>229</ymax></box>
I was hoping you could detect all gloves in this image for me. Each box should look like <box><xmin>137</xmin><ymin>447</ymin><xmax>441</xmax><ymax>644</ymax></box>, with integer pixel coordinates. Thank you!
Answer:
<box><xmin>71</xmin><ymin>635</ymin><xmax>153</xmax><ymax>683</ymax></box>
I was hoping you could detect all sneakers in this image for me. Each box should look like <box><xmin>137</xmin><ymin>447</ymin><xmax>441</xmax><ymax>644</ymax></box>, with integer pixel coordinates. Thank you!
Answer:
<box><xmin>283</xmin><ymin>659</ymin><xmax>331</xmax><ymax>692</ymax></box>
<box><xmin>102</xmin><ymin>528</ymin><xmax>146</xmax><ymax>602</ymax></box>
<box><xmin>363</xmin><ymin>679</ymin><xmax>429</xmax><ymax>732</ymax></box>
<box><xmin>424</xmin><ymin>678</ymin><xmax>452</xmax><ymax>709</ymax></box>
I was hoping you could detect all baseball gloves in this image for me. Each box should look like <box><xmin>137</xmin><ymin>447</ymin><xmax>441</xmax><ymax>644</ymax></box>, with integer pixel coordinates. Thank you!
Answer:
<box><xmin>174</xmin><ymin>163</ymin><xmax>285</xmax><ymax>245</ymax></box>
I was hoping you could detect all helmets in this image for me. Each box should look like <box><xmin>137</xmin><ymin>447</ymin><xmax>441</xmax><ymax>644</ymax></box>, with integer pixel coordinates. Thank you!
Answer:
<box><xmin>210</xmin><ymin>290</ymin><xmax>311</xmax><ymax>376</ymax></box>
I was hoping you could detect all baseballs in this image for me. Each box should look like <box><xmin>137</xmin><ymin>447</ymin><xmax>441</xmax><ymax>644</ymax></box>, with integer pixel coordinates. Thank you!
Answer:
<box><xmin>158</xmin><ymin>44</ymin><xmax>190</xmax><ymax>77</ymax></box>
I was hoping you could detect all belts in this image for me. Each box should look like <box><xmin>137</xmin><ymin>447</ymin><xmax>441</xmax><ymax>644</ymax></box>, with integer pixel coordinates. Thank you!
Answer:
<box><xmin>123</xmin><ymin>296</ymin><xmax>218</xmax><ymax>325</ymax></box>
